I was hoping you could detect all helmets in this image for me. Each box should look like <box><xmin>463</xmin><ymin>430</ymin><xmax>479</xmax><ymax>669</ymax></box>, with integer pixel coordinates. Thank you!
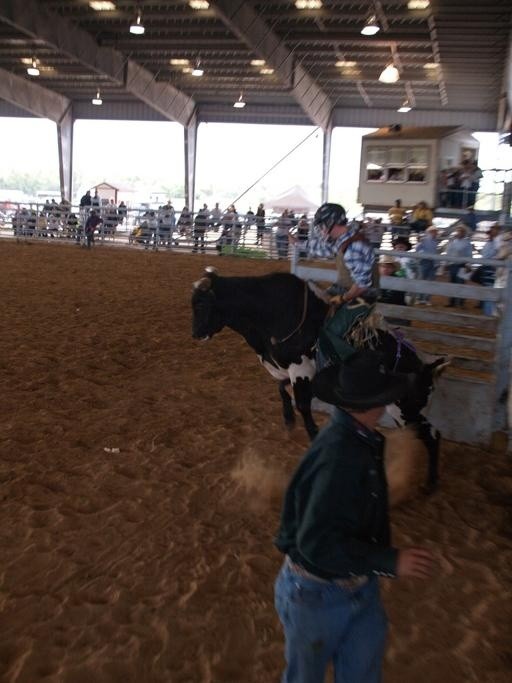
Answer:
<box><xmin>314</xmin><ymin>203</ymin><xmax>347</xmax><ymax>241</ymax></box>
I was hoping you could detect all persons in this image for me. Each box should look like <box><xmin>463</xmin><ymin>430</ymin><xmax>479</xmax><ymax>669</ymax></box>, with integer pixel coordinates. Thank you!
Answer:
<box><xmin>11</xmin><ymin>191</ymin><xmax>313</xmax><ymax>260</ymax></box>
<box><xmin>274</xmin><ymin>349</ymin><xmax>438</xmax><ymax>682</ymax></box>
<box><xmin>361</xmin><ymin>159</ymin><xmax>512</xmax><ymax>326</ymax></box>
<box><xmin>288</xmin><ymin>203</ymin><xmax>380</xmax><ymax>361</ymax></box>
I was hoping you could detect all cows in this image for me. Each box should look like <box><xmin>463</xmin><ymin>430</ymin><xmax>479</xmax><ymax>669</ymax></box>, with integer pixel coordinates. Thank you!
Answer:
<box><xmin>189</xmin><ymin>265</ymin><xmax>510</xmax><ymax>497</ymax></box>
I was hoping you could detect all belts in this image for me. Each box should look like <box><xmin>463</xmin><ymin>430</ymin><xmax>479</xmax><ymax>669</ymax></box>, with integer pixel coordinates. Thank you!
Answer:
<box><xmin>286</xmin><ymin>558</ymin><xmax>368</xmax><ymax>590</ymax></box>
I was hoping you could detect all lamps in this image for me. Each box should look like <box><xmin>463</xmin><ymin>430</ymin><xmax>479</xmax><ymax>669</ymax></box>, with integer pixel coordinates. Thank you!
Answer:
<box><xmin>26</xmin><ymin>0</ymin><xmax>441</xmax><ymax>114</ymax></box>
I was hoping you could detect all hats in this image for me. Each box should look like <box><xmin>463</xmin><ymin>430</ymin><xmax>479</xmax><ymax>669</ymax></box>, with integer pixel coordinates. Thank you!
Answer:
<box><xmin>376</xmin><ymin>254</ymin><xmax>401</xmax><ymax>272</ymax></box>
<box><xmin>311</xmin><ymin>348</ymin><xmax>417</xmax><ymax>410</ymax></box>
<box><xmin>391</xmin><ymin>237</ymin><xmax>412</xmax><ymax>250</ymax></box>
